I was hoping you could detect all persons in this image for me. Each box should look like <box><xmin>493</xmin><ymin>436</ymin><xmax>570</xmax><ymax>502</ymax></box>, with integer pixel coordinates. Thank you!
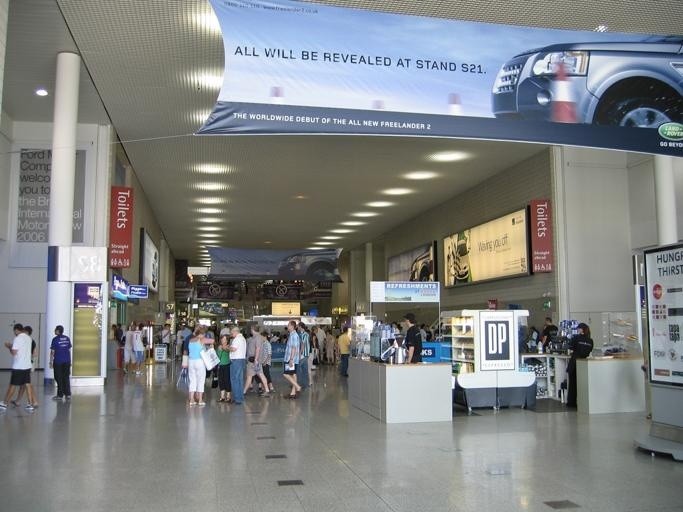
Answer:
<box><xmin>527</xmin><ymin>316</ymin><xmax>593</xmax><ymax>407</ymax></box>
<box><xmin>9</xmin><ymin>325</ymin><xmax>40</xmax><ymax>409</ymax></box>
<box><xmin>49</xmin><ymin>325</ymin><xmax>72</xmax><ymax>400</ymax></box>
<box><xmin>0</xmin><ymin>323</ymin><xmax>36</xmax><ymax>411</ymax></box>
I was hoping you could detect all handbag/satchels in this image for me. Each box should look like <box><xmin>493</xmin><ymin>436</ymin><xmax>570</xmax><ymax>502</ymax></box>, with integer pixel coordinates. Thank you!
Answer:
<box><xmin>200</xmin><ymin>346</ymin><xmax>221</xmax><ymax>371</ymax></box>
<box><xmin>248</xmin><ymin>356</ymin><xmax>255</xmax><ymax>363</ymax></box>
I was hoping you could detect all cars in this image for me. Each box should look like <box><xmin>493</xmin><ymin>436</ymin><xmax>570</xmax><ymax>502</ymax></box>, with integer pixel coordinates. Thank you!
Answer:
<box><xmin>408</xmin><ymin>244</ymin><xmax>434</xmax><ymax>282</ymax></box>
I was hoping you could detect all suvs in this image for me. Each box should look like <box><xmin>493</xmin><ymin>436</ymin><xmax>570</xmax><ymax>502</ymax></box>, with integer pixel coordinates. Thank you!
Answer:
<box><xmin>277</xmin><ymin>249</ymin><xmax>343</xmax><ymax>282</ymax></box>
<box><xmin>488</xmin><ymin>33</ymin><xmax>683</xmax><ymax>130</ymax></box>
<box><xmin>446</xmin><ymin>238</ymin><xmax>472</xmax><ymax>281</ymax></box>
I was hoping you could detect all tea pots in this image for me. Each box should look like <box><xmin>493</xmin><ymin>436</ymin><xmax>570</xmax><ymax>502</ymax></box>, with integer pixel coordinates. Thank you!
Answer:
<box><xmin>394</xmin><ymin>347</ymin><xmax>406</xmax><ymax>365</ymax></box>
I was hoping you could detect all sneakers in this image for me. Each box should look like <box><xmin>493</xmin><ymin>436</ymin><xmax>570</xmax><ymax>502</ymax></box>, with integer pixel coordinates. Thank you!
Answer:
<box><xmin>0</xmin><ymin>399</ymin><xmax>9</xmax><ymax>410</ymax></box>
<box><xmin>215</xmin><ymin>386</ymin><xmax>302</xmax><ymax>403</ymax></box>
<box><xmin>11</xmin><ymin>400</ymin><xmax>20</xmax><ymax>408</ymax></box>
<box><xmin>123</xmin><ymin>367</ymin><xmax>144</xmax><ymax>376</ymax></box>
<box><xmin>189</xmin><ymin>401</ymin><xmax>206</xmax><ymax>407</ymax></box>
<box><xmin>52</xmin><ymin>394</ymin><xmax>72</xmax><ymax>402</ymax></box>
<box><xmin>24</xmin><ymin>402</ymin><xmax>38</xmax><ymax>411</ymax></box>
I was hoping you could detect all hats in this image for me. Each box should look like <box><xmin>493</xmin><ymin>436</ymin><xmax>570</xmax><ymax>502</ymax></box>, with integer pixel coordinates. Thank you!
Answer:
<box><xmin>403</xmin><ymin>313</ymin><xmax>417</xmax><ymax>324</ymax></box>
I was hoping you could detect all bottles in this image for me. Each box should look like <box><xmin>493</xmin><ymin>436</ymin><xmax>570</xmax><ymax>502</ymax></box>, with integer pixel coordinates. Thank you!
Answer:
<box><xmin>537</xmin><ymin>340</ymin><xmax>543</xmax><ymax>355</ymax></box>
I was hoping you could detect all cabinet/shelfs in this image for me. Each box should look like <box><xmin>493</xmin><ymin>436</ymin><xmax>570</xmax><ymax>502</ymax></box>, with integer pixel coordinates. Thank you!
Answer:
<box><xmin>521</xmin><ymin>354</ymin><xmax>572</xmax><ymax>404</ymax></box>
<box><xmin>440</xmin><ymin>309</ymin><xmax>480</xmax><ymax>376</ymax></box>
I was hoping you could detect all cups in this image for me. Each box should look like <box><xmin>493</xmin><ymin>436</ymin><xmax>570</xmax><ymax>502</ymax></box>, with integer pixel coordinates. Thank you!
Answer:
<box><xmin>387</xmin><ymin>354</ymin><xmax>394</xmax><ymax>367</ymax></box>
